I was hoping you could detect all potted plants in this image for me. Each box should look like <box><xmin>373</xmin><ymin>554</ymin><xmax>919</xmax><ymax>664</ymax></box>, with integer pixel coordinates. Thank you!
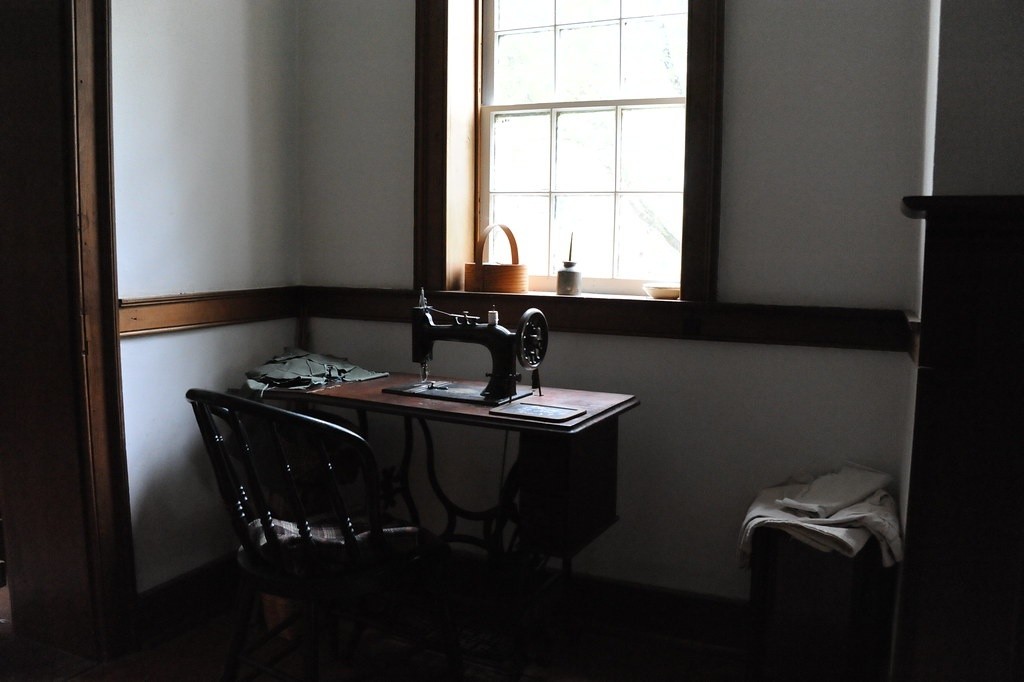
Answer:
<box><xmin>556</xmin><ymin>231</ymin><xmax>581</xmax><ymax>295</ymax></box>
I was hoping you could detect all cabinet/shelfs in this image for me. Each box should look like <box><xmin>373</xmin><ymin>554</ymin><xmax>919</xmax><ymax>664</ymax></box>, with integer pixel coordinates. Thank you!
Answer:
<box><xmin>887</xmin><ymin>196</ymin><xmax>1024</xmax><ymax>682</ymax></box>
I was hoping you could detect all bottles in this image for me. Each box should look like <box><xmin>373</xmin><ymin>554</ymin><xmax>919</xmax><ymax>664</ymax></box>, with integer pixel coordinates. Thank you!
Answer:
<box><xmin>556</xmin><ymin>261</ymin><xmax>580</xmax><ymax>294</ymax></box>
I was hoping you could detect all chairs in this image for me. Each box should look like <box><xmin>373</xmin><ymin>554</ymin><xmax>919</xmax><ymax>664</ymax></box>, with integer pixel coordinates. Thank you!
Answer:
<box><xmin>186</xmin><ymin>390</ymin><xmax>464</xmax><ymax>682</ymax></box>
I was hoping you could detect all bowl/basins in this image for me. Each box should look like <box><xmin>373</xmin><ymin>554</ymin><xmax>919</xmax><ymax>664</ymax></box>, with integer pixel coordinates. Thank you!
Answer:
<box><xmin>642</xmin><ymin>284</ymin><xmax>679</xmax><ymax>299</ymax></box>
